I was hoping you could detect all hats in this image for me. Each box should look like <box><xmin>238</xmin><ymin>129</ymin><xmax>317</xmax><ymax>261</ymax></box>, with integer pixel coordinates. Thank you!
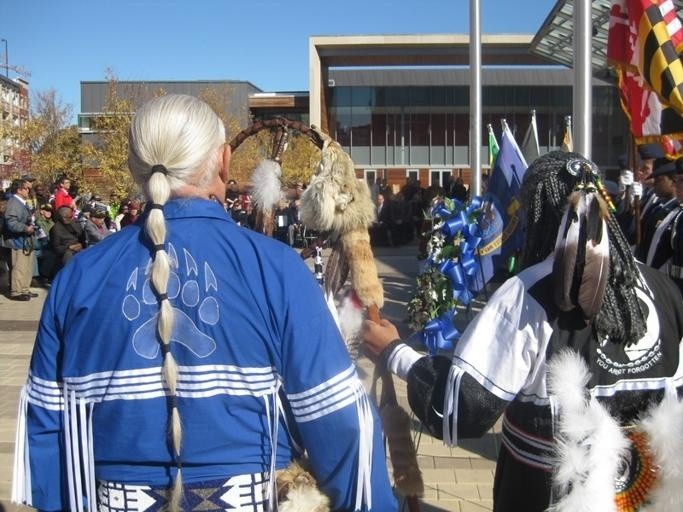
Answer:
<box><xmin>646</xmin><ymin>157</ymin><xmax>683</xmax><ymax>178</ymax></box>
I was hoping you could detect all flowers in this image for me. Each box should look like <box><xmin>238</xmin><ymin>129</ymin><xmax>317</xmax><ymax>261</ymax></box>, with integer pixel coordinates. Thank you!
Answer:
<box><xmin>403</xmin><ymin>195</ymin><xmax>461</xmax><ymax>337</ymax></box>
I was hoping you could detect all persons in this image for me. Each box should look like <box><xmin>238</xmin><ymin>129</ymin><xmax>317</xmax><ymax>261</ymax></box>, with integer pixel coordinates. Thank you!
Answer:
<box><xmin>11</xmin><ymin>94</ymin><xmax>399</xmax><ymax>512</ymax></box>
<box><xmin>357</xmin><ymin>150</ymin><xmax>683</xmax><ymax>512</ymax></box>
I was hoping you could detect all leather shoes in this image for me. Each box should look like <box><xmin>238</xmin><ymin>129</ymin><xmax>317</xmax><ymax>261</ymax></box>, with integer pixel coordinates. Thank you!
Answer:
<box><xmin>9</xmin><ymin>292</ymin><xmax>38</xmax><ymax>300</ymax></box>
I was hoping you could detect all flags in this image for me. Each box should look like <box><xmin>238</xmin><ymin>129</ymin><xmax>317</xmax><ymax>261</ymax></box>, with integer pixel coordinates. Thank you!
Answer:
<box><xmin>457</xmin><ymin>110</ymin><xmax>573</xmax><ymax>307</ymax></box>
<box><xmin>607</xmin><ymin>0</ymin><xmax>683</xmax><ymax>161</ymax></box>
